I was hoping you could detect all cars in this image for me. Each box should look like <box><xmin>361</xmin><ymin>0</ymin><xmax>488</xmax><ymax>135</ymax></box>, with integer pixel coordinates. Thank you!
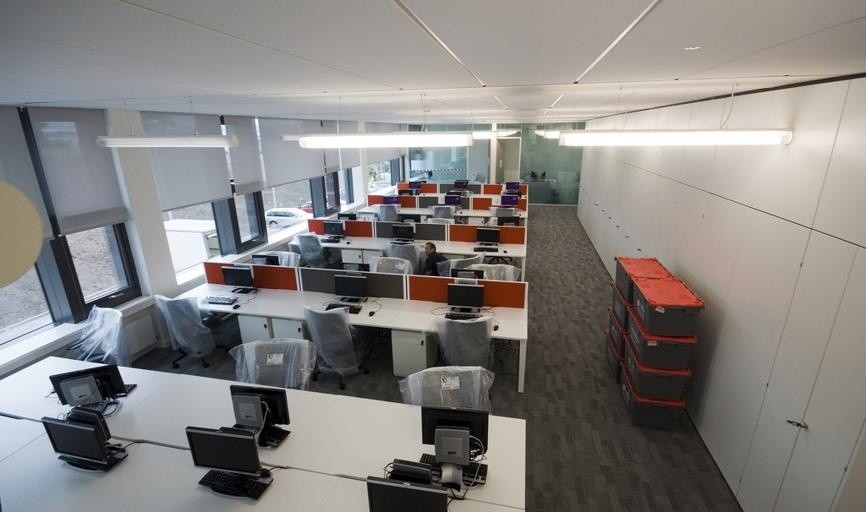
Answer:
<box><xmin>299</xmin><ymin>200</ymin><xmax>318</xmax><ymax>213</ymax></box>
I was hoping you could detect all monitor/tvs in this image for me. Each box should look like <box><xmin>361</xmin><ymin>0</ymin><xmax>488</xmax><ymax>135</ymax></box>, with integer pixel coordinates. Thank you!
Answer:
<box><xmin>382</xmin><ymin>179</ymin><xmax>520</xmax><ymax>246</ymax></box>
<box><xmin>41</xmin><ymin>364</ymin><xmax>130</xmax><ymax>474</ymax></box>
<box><xmin>323</xmin><ymin>213</ymin><xmax>357</xmax><ymax>239</ymax></box>
<box><xmin>183</xmin><ymin>385</ymin><xmax>290</xmax><ymax>477</ymax></box>
<box><xmin>447</xmin><ymin>268</ymin><xmax>485</xmax><ymax>312</ymax></box>
<box><xmin>366</xmin><ymin>404</ymin><xmax>490</xmax><ymax>512</ymax></box>
<box><xmin>220</xmin><ymin>254</ymin><xmax>370</xmax><ymax>302</ymax></box>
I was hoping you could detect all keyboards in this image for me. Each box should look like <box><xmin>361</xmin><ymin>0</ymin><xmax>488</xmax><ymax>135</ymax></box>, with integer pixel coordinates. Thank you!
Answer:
<box><xmin>208</xmin><ymin>297</ymin><xmax>237</xmax><ymax>305</ymax></box>
<box><xmin>474</xmin><ymin>248</ymin><xmax>498</xmax><ymax>252</ymax></box>
<box><xmin>320</xmin><ymin>239</ymin><xmax>339</xmax><ymax>243</ymax></box>
<box><xmin>124</xmin><ymin>385</ymin><xmax>137</xmax><ymax>394</ymax></box>
<box><xmin>445</xmin><ymin>312</ymin><xmax>480</xmax><ymax>320</ymax></box>
<box><xmin>199</xmin><ymin>470</ymin><xmax>273</xmax><ymax>501</ymax></box>
<box><xmin>325</xmin><ymin>303</ymin><xmax>363</xmax><ymax>314</ymax></box>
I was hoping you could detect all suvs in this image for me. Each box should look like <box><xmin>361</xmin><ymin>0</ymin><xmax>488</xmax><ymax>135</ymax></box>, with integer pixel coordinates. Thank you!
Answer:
<box><xmin>369</xmin><ymin>172</ymin><xmax>392</xmax><ymax>190</ymax></box>
<box><xmin>265</xmin><ymin>207</ymin><xmax>312</xmax><ymax>226</ymax></box>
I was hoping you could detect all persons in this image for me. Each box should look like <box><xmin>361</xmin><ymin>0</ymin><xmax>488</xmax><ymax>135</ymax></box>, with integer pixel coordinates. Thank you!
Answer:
<box><xmin>419</xmin><ymin>241</ymin><xmax>448</xmax><ymax>277</ymax></box>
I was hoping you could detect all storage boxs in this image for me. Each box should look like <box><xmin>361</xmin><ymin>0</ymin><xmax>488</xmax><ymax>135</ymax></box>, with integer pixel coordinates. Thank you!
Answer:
<box><xmin>604</xmin><ymin>253</ymin><xmax>707</xmax><ymax>434</ymax></box>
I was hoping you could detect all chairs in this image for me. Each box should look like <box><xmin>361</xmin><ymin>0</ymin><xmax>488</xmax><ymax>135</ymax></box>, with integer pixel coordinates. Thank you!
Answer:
<box><xmin>64</xmin><ymin>303</ymin><xmax>129</xmax><ymax>369</ymax></box>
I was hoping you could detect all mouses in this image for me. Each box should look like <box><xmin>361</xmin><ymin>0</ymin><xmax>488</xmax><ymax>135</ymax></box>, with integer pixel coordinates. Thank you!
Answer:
<box><xmin>234</xmin><ymin>305</ymin><xmax>240</xmax><ymax>309</ymax></box>
<box><xmin>494</xmin><ymin>325</ymin><xmax>498</xmax><ymax>330</ymax></box>
<box><xmin>347</xmin><ymin>242</ymin><xmax>349</xmax><ymax>244</ymax></box>
<box><xmin>369</xmin><ymin>312</ymin><xmax>375</xmax><ymax>316</ymax></box>
<box><xmin>504</xmin><ymin>250</ymin><xmax>507</xmax><ymax>253</ymax></box>
